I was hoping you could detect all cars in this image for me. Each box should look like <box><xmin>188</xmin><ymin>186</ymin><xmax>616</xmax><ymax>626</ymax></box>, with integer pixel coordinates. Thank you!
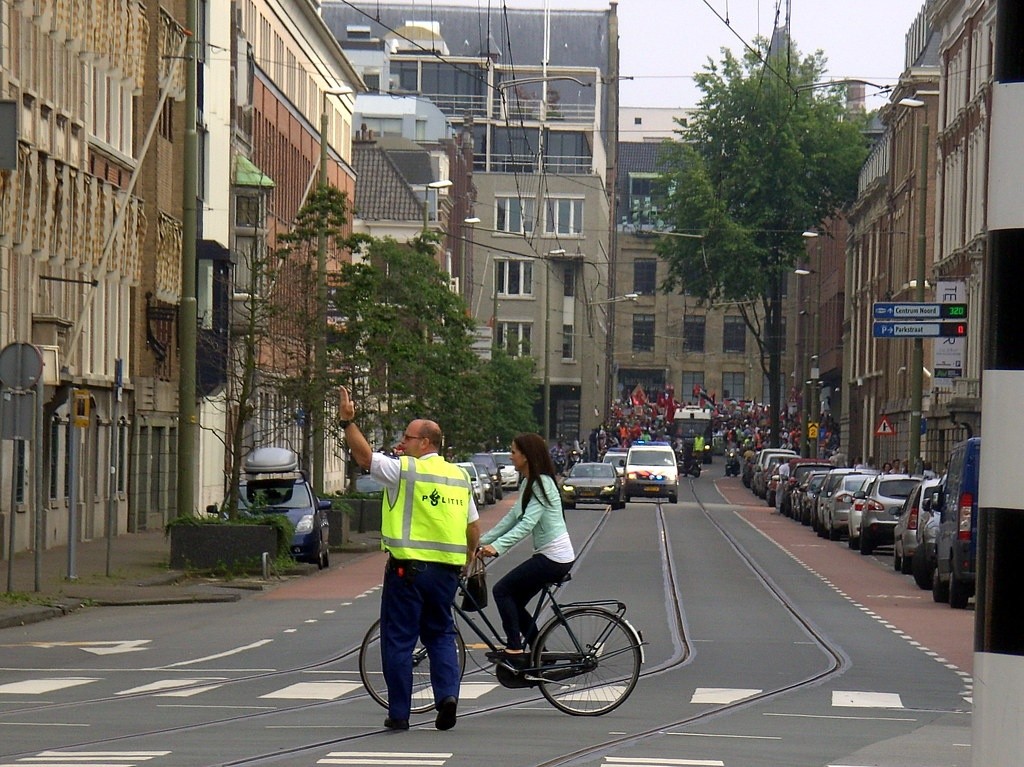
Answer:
<box><xmin>750</xmin><ymin>449</ymin><xmax>875</xmax><ymax>549</ymax></box>
<box><xmin>561</xmin><ymin>462</ymin><xmax>625</xmax><ymax>511</ymax></box>
<box><xmin>452</xmin><ymin>452</ymin><xmax>520</xmax><ymax>511</ymax></box>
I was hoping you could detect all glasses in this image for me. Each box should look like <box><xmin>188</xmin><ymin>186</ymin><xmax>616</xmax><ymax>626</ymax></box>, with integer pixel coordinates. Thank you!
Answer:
<box><xmin>406</xmin><ymin>435</ymin><xmax>425</xmax><ymax>440</ymax></box>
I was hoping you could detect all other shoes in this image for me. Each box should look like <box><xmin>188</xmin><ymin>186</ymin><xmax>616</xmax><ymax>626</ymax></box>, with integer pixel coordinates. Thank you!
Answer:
<box><xmin>771</xmin><ymin>510</ymin><xmax>780</xmax><ymax>516</ymax></box>
<box><xmin>384</xmin><ymin>717</ymin><xmax>409</xmax><ymax>729</ymax></box>
<box><xmin>485</xmin><ymin>649</ymin><xmax>524</xmax><ymax>658</ymax></box>
<box><xmin>435</xmin><ymin>696</ymin><xmax>457</xmax><ymax>730</ymax></box>
<box><xmin>541</xmin><ymin>659</ymin><xmax>556</xmax><ymax>665</ymax></box>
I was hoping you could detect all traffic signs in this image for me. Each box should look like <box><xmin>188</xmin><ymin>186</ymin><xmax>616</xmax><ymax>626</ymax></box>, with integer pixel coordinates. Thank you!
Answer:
<box><xmin>873</xmin><ymin>301</ymin><xmax>968</xmax><ymax>338</ymax></box>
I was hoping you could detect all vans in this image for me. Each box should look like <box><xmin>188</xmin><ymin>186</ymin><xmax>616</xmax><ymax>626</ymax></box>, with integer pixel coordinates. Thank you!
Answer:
<box><xmin>933</xmin><ymin>436</ymin><xmax>979</xmax><ymax>610</ymax></box>
<box><xmin>672</xmin><ymin>405</ymin><xmax>713</xmax><ymax>464</ymax></box>
<box><xmin>621</xmin><ymin>441</ymin><xmax>683</xmax><ymax>504</ymax></box>
<box><xmin>602</xmin><ymin>447</ymin><xmax>628</xmax><ymax>484</ymax></box>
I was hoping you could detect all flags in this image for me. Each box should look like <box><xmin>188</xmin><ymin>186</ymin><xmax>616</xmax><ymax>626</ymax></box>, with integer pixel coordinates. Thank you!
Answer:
<box><xmin>632</xmin><ymin>384</ymin><xmax>646</xmax><ymax>405</ymax></box>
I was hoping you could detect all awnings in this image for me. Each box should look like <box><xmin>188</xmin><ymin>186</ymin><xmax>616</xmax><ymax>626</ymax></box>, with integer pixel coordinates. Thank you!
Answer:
<box><xmin>543</xmin><ymin>252</ymin><xmax>585</xmax><ymax>264</ymax></box>
<box><xmin>235</xmin><ymin>154</ymin><xmax>276</xmax><ymax>187</ymax></box>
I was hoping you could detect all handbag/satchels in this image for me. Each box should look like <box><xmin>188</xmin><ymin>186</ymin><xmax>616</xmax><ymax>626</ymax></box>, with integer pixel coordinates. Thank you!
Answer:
<box><xmin>461</xmin><ymin>550</ymin><xmax>488</xmax><ymax>611</ymax></box>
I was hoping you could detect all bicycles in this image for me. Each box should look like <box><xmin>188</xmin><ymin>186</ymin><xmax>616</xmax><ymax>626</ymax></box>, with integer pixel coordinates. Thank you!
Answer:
<box><xmin>359</xmin><ymin>542</ymin><xmax>650</xmax><ymax>715</ymax></box>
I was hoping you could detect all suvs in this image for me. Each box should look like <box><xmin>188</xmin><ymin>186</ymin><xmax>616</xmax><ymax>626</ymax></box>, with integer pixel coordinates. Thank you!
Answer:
<box><xmin>205</xmin><ymin>471</ymin><xmax>332</xmax><ymax>570</ymax></box>
<box><xmin>859</xmin><ymin>472</ymin><xmax>947</xmax><ymax>589</ymax></box>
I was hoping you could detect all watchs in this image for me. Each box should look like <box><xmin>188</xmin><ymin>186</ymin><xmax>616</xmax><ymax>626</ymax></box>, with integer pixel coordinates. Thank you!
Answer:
<box><xmin>339</xmin><ymin>417</ymin><xmax>356</xmax><ymax>429</ymax></box>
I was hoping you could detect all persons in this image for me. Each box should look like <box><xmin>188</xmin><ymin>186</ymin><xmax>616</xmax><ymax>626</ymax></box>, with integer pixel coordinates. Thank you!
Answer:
<box><xmin>769</xmin><ymin>457</ymin><xmax>790</xmax><ymax>515</ymax></box>
<box><xmin>724</xmin><ymin>437</ymin><xmax>737</xmax><ymax>476</ymax></box>
<box><xmin>550</xmin><ymin>396</ymin><xmax>705</xmax><ymax>478</ymax></box>
<box><xmin>377</xmin><ymin>440</ymin><xmax>404</xmax><ymax>456</ymax></box>
<box><xmin>854</xmin><ymin>456</ymin><xmax>950</xmax><ymax>480</ymax></box>
<box><xmin>447</xmin><ymin>446</ymin><xmax>456</xmax><ymax>461</ymax></box>
<box><xmin>339</xmin><ymin>385</ymin><xmax>482</xmax><ymax>730</ymax></box>
<box><xmin>714</xmin><ymin>398</ymin><xmax>845</xmax><ymax>473</ymax></box>
<box><xmin>483</xmin><ymin>439</ymin><xmax>492</xmax><ymax>453</ymax></box>
<box><xmin>480</xmin><ymin>434</ymin><xmax>576</xmax><ymax>662</ymax></box>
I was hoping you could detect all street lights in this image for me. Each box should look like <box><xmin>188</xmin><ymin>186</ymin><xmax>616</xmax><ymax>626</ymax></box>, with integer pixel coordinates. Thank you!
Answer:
<box><xmin>460</xmin><ymin>216</ymin><xmax>481</xmax><ymax>293</ymax></box>
<box><xmin>794</xmin><ymin>231</ymin><xmax>822</xmax><ymax>458</ymax></box>
<box><xmin>544</xmin><ymin>247</ymin><xmax>567</xmax><ymax>444</ymax></box>
<box><xmin>897</xmin><ymin>97</ymin><xmax>934</xmax><ymax>473</ymax></box>
<box><xmin>422</xmin><ymin>179</ymin><xmax>452</xmax><ymax>343</ymax></box>
<box><xmin>312</xmin><ymin>83</ymin><xmax>358</xmax><ymax>495</ymax></box>
